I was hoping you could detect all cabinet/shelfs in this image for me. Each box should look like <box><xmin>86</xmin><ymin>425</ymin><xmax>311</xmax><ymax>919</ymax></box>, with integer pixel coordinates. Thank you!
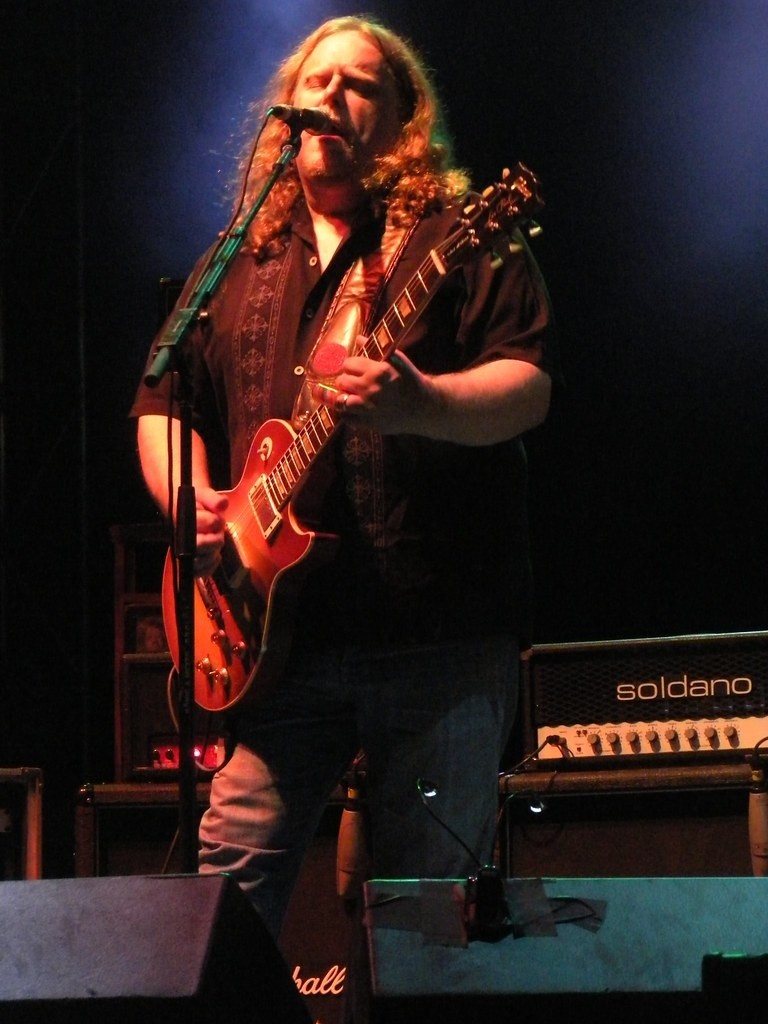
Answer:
<box><xmin>112</xmin><ymin>523</ymin><xmax>228</xmax><ymax>780</ymax></box>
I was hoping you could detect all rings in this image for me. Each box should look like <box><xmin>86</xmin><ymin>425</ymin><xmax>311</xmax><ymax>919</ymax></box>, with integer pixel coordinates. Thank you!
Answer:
<box><xmin>342</xmin><ymin>392</ymin><xmax>349</xmax><ymax>411</ymax></box>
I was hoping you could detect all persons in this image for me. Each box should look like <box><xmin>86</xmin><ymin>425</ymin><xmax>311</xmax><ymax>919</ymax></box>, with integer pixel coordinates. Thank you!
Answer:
<box><xmin>128</xmin><ymin>14</ymin><xmax>556</xmax><ymax>1024</ymax></box>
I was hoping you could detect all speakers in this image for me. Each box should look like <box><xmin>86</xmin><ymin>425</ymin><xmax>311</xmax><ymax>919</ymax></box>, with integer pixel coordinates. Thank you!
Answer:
<box><xmin>106</xmin><ymin>521</ymin><xmax>182</xmax><ymax>783</ymax></box>
<box><xmin>0</xmin><ymin>764</ymin><xmax>768</xmax><ymax>1024</ymax></box>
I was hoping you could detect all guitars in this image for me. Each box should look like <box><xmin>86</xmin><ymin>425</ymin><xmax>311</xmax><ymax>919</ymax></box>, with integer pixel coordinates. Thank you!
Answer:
<box><xmin>150</xmin><ymin>158</ymin><xmax>557</xmax><ymax>717</ymax></box>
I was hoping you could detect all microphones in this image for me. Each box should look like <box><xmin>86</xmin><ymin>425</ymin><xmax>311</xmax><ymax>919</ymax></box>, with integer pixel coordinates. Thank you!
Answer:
<box><xmin>271</xmin><ymin>107</ymin><xmax>330</xmax><ymax>137</ymax></box>
<box><xmin>332</xmin><ymin>770</ymin><xmax>364</xmax><ymax>900</ymax></box>
